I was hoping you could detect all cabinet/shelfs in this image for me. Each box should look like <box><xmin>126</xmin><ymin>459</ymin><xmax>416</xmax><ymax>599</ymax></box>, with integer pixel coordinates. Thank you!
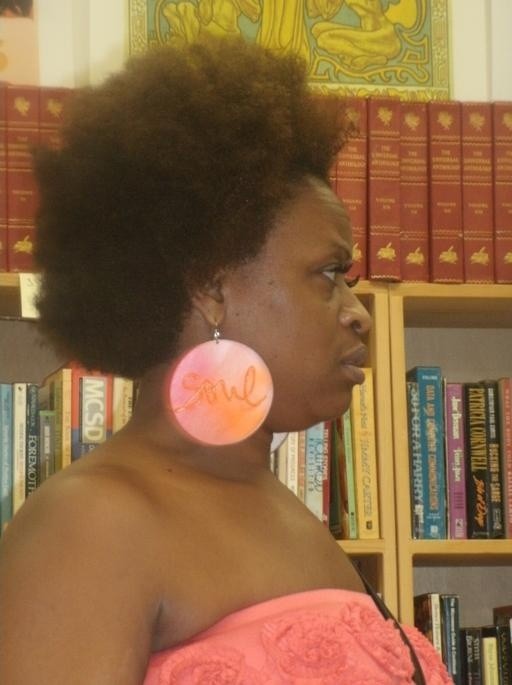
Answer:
<box><xmin>390</xmin><ymin>285</ymin><xmax>512</xmax><ymax>629</ymax></box>
<box><xmin>0</xmin><ymin>271</ymin><xmax>398</xmax><ymax>619</ymax></box>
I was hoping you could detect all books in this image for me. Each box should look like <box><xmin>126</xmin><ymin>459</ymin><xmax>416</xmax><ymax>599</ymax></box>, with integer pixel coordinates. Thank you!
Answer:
<box><xmin>2</xmin><ymin>360</ymin><xmax>136</xmax><ymax>532</ymax></box>
<box><xmin>444</xmin><ymin>379</ymin><xmax>512</xmax><ymax>539</ymax></box>
<box><xmin>299</xmin><ymin>96</ymin><xmax>462</xmax><ymax>284</ymax></box>
<box><xmin>412</xmin><ymin>592</ymin><xmax>451</xmax><ymax>676</ymax></box>
<box><xmin>1</xmin><ymin>85</ymin><xmax>82</xmax><ymax>275</ymax></box>
<box><xmin>407</xmin><ymin>366</ymin><xmax>448</xmax><ymax>540</ymax></box>
<box><xmin>267</xmin><ymin>366</ymin><xmax>380</xmax><ymax>538</ymax></box>
<box><xmin>452</xmin><ymin>594</ymin><xmax>512</xmax><ymax>685</ymax></box>
<box><xmin>460</xmin><ymin>101</ymin><xmax>495</xmax><ymax>284</ymax></box>
<box><xmin>489</xmin><ymin>100</ymin><xmax>512</xmax><ymax>284</ymax></box>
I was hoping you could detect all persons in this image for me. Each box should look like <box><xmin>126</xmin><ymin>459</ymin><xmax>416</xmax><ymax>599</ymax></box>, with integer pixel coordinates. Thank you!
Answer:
<box><xmin>3</xmin><ymin>31</ymin><xmax>450</xmax><ymax>684</ymax></box>
